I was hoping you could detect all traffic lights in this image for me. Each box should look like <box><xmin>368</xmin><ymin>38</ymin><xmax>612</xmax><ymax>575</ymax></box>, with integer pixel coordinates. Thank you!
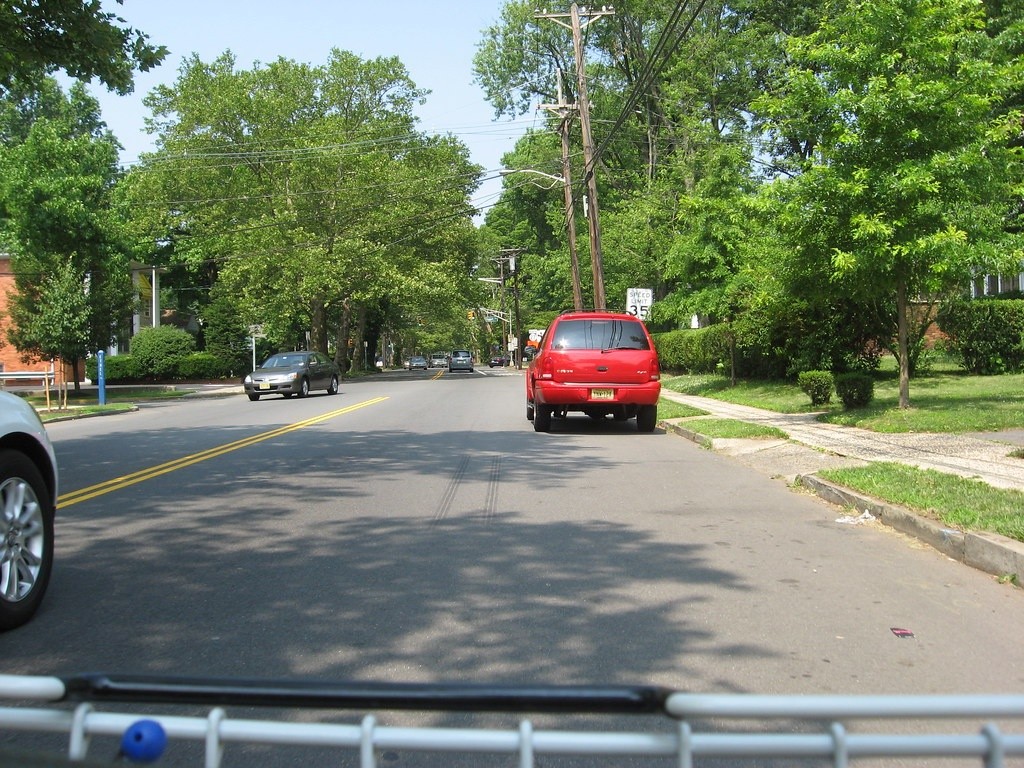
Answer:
<box><xmin>467</xmin><ymin>311</ymin><xmax>473</xmax><ymax>321</ymax></box>
<box><xmin>416</xmin><ymin>318</ymin><xmax>425</xmax><ymax>326</ymax></box>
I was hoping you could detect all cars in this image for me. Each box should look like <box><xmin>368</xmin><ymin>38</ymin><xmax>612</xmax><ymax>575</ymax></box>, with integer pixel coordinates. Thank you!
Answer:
<box><xmin>376</xmin><ymin>358</ymin><xmax>388</xmax><ymax>368</ymax></box>
<box><xmin>403</xmin><ymin>357</ymin><xmax>413</xmax><ymax>369</ymax></box>
<box><xmin>429</xmin><ymin>354</ymin><xmax>448</xmax><ymax>368</ymax></box>
<box><xmin>244</xmin><ymin>351</ymin><xmax>342</xmax><ymax>402</ymax></box>
<box><xmin>488</xmin><ymin>353</ymin><xmax>515</xmax><ymax>368</ymax></box>
<box><xmin>0</xmin><ymin>386</ymin><xmax>60</xmax><ymax>631</ymax></box>
<box><xmin>409</xmin><ymin>356</ymin><xmax>428</xmax><ymax>371</ymax></box>
<box><xmin>448</xmin><ymin>349</ymin><xmax>475</xmax><ymax>373</ymax></box>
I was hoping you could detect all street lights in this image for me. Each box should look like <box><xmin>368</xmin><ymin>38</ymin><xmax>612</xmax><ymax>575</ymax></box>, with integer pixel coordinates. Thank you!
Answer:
<box><xmin>499</xmin><ymin>169</ymin><xmax>583</xmax><ymax>311</ymax></box>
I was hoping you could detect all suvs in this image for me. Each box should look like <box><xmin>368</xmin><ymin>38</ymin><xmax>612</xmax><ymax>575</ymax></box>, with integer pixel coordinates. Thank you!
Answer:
<box><xmin>525</xmin><ymin>307</ymin><xmax>662</xmax><ymax>432</ymax></box>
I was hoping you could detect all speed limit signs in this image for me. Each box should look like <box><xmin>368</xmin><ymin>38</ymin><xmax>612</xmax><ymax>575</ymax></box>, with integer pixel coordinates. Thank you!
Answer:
<box><xmin>626</xmin><ymin>287</ymin><xmax>653</xmax><ymax>322</ymax></box>
